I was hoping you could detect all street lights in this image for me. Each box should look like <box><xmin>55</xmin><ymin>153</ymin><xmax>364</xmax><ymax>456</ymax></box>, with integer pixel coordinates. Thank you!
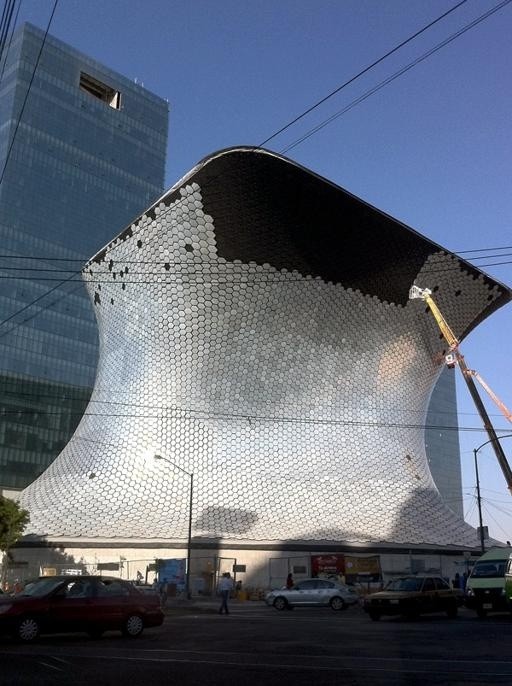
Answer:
<box><xmin>152</xmin><ymin>454</ymin><xmax>193</xmax><ymax>597</ymax></box>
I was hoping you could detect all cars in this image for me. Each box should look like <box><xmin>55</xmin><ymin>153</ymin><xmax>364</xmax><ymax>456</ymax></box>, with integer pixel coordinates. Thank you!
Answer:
<box><xmin>365</xmin><ymin>576</ymin><xmax>466</xmax><ymax>620</ymax></box>
<box><xmin>264</xmin><ymin>578</ymin><xmax>361</xmax><ymax>611</ymax></box>
<box><xmin>0</xmin><ymin>576</ymin><xmax>165</xmax><ymax>642</ymax></box>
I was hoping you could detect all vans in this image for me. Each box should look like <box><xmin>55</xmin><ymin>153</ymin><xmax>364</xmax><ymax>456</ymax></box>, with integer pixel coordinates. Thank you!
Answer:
<box><xmin>466</xmin><ymin>547</ymin><xmax>512</xmax><ymax>617</ymax></box>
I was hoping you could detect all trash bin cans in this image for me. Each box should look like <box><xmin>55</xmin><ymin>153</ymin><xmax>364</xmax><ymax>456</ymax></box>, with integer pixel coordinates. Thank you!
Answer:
<box><xmin>192</xmin><ymin>578</ymin><xmax>205</xmax><ymax>597</ymax></box>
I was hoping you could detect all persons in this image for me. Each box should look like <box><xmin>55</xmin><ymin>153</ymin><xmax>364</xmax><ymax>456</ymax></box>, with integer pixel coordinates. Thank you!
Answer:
<box><xmin>462</xmin><ymin>571</ymin><xmax>470</xmax><ymax>608</ymax></box>
<box><xmin>454</xmin><ymin>572</ymin><xmax>466</xmax><ymax>588</ymax></box>
<box><xmin>159</xmin><ymin>577</ymin><xmax>170</xmax><ymax>620</ymax></box>
<box><xmin>134</xmin><ymin>571</ymin><xmax>144</xmax><ymax>586</ymax></box>
<box><xmin>176</xmin><ymin>579</ymin><xmax>186</xmax><ymax>601</ymax></box>
<box><xmin>151</xmin><ymin>578</ymin><xmax>159</xmax><ymax>590</ymax></box>
<box><xmin>217</xmin><ymin>571</ymin><xmax>233</xmax><ymax>614</ymax></box>
<box><xmin>286</xmin><ymin>572</ymin><xmax>294</xmax><ymax>587</ymax></box>
<box><xmin>226</xmin><ymin>572</ymin><xmax>236</xmax><ymax>602</ymax></box>
<box><xmin>337</xmin><ymin>571</ymin><xmax>346</xmax><ymax>585</ymax></box>
<box><xmin>236</xmin><ymin>580</ymin><xmax>241</xmax><ymax>597</ymax></box>
